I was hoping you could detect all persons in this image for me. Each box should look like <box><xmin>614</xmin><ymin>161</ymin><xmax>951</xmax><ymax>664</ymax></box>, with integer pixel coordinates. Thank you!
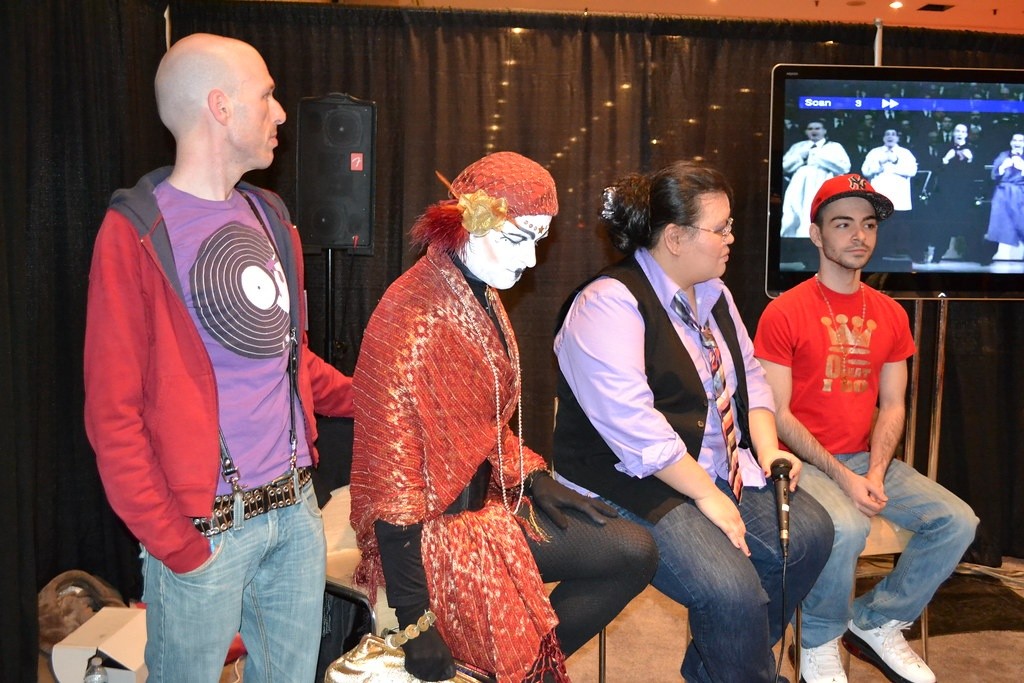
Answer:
<box><xmin>81</xmin><ymin>31</ymin><xmax>353</xmax><ymax>683</ymax></box>
<box><xmin>754</xmin><ymin>174</ymin><xmax>980</xmax><ymax>683</ymax></box>
<box><xmin>784</xmin><ymin>75</ymin><xmax>1024</xmax><ymax>261</ymax></box>
<box><xmin>551</xmin><ymin>160</ymin><xmax>834</xmax><ymax>683</ymax></box>
<box><xmin>350</xmin><ymin>152</ymin><xmax>657</xmax><ymax>683</ymax></box>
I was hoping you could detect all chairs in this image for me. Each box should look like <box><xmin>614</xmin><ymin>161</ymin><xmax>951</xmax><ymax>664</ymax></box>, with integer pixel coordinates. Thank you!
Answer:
<box><xmin>315</xmin><ymin>574</ymin><xmax>380</xmax><ymax>683</ymax></box>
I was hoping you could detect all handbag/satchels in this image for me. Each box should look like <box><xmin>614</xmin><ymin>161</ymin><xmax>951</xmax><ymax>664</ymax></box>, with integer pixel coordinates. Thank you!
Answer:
<box><xmin>322</xmin><ymin>610</ymin><xmax>484</xmax><ymax>683</ymax></box>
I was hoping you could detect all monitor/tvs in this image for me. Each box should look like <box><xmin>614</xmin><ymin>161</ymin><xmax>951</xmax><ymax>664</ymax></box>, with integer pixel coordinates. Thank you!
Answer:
<box><xmin>767</xmin><ymin>66</ymin><xmax>1024</xmax><ymax>299</ymax></box>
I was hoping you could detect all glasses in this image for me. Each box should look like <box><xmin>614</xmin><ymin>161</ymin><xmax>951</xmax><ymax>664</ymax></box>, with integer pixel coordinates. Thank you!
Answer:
<box><xmin>689</xmin><ymin>218</ymin><xmax>733</xmax><ymax>236</ymax></box>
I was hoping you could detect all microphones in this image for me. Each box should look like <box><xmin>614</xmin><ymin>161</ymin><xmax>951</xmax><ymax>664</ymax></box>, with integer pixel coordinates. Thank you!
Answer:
<box><xmin>771</xmin><ymin>457</ymin><xmax>793</xmax><ymax>555</ymax></box>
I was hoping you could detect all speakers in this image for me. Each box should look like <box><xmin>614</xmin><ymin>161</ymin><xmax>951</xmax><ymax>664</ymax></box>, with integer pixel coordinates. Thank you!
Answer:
<box><xmin>272</xmin><ymin>91</ymin><xmax>377</xmax><ymax>257</ymax></box>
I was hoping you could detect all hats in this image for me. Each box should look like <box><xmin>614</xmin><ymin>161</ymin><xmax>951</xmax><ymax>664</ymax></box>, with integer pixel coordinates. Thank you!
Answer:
<box><xmin>410</xmin><ymin>152</ymin><xmax>559</xmax><ymax>257</ymax></box>
<box><xmin>810</xmin><ymin>173</ymin><xmax>894</xmax><ymax>222</ymax></box>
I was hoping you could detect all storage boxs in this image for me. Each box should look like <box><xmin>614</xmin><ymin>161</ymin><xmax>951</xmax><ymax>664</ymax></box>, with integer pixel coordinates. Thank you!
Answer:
<box><xmin>51</xmin><ymin>605</ymin><xmax>148</xmax><ymax>683</ymax></box>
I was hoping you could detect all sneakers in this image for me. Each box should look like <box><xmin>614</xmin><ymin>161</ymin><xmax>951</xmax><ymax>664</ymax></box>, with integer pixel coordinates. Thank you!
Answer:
<box><xmin>791</xmin><ymin>633</ymin><xmax>849</xmax><ymax>683</ymax></box>
<box><xmin>841</xmin><ymin>616</ymin><xmax>937</xmax><ymax>683</ymax></box>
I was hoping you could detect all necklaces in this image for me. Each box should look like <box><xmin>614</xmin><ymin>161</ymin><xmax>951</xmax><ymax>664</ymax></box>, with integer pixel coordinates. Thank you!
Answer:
<box><xmin>816</xmin><ymin>273</ymin><xmax>866</xmax><ymax>387</ymax></box>
<box><xmin>429</xmin><ymin>254</ymin><xmax>524</xmax><ymax>514</ymax></box>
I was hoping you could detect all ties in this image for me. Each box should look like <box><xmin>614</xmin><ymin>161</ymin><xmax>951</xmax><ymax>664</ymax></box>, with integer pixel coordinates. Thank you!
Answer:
<box><xmin>674</xmin><ymin>298</ymin><xmax>743</xmax><ymax>506</ymax></box>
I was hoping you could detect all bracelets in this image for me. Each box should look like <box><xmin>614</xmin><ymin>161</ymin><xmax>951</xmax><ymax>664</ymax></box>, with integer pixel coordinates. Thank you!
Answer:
<box><xmin>387</xmin><ymin>609</ymin><xmax>436</xmax><ymax>650</ymax></box>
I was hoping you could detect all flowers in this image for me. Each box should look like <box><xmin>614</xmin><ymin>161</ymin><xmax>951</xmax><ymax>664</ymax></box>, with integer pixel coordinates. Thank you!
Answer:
<box><xmin>457</xmin><ymin>190</ymin><xmax>508</xmax><ymax>237</ymax></box>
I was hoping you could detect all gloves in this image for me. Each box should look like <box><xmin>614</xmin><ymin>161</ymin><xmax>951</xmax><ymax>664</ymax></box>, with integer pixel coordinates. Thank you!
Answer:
<box><xmin>399</xmin><ymin>601</ymin><xmax>455</xmax><ymax>683</ymax></box>
<box><xmin>528</xmin><ymin>471</ymin><xmax>620</xmax><ymax>530</ymax></box>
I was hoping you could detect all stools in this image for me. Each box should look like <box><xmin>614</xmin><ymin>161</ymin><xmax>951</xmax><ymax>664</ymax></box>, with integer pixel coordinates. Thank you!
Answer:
<box><xmin>794</xmin><ymin>515</ymin><xmax>929</xmax><ymax>683</ymax></box>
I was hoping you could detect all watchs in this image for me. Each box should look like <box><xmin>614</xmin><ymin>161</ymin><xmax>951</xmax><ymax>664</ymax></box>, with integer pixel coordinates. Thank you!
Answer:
<box><xmin>525</xmin><ymin>470</ymin><xmax>545</xmax><ymax>493</ymax></box>
